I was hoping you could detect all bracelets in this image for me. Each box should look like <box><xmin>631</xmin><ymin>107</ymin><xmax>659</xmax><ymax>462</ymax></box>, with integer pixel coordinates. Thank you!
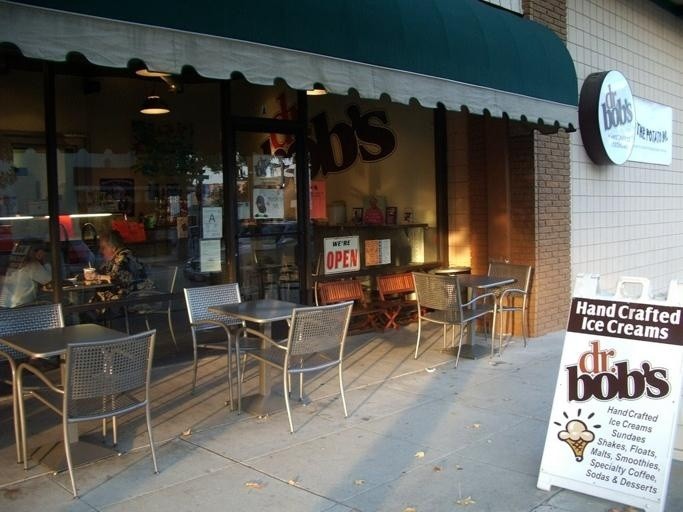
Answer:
<box><xmin>97</xmin><ymin>274</ymin><xmax>104</xmax><ymax>281</ymax></box>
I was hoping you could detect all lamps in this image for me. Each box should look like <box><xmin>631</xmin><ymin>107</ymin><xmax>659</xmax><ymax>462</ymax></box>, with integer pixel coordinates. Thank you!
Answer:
<box><xmin>139</xmin><ymin>93</ymin><xmax>170</xmax><ymax>114</ymax></box>
<box><xmin>133</xmin><ymin>59</ymin><xmax>173</xmax><ymax>79</ymax></box>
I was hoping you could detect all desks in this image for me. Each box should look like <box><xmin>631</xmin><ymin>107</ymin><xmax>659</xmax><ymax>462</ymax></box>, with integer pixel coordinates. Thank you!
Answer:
<box><xmin>41</xmin><ymin>279</ymin><xmax>122</xmax><ymax>326</ymax></box>
<box><xmin>207</xmin><ymin>301</ymin><xmax>314</xmax><ymax>418</ymax></box>
<box><xmin>0</xmin><ymin>322</ymin><xmax>130</xmax><ymax>475</ymax></box>
<box><xmin>440</xmin><ymin>273</ymin><xmax>515</xmax><ymax>360</ymax></box>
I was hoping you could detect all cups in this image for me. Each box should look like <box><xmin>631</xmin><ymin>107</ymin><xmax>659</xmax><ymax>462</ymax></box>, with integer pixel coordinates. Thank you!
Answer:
<box><xmin>83</xmin><ymin>268</ymin><xmax>95</xmax><ymax>286</ymax></box>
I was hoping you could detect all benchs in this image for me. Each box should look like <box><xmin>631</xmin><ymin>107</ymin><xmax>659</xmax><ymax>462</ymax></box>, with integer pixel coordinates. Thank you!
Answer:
<box><xmin>317</xmin><ymin>279</ymin><xmax>384</xmax><ymax>334</ymax></box>
<box><xmin>377</xmin><ymin>273</ymin><xmax>425</xmax><ymax>328</ymax></box>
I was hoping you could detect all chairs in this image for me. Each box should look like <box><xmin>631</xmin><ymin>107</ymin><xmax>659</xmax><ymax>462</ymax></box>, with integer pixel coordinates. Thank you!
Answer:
<box><xmin>233</xmin><ymin>299</ymin><xmax>354</xmax><ymax>434</ymax></box>
<box><xmin>123</xmin><ymin>264</ymin><xmax>181</xmax><ymax>352</ymax></box>
<box><xmin>15</xmin><ymin>327</ymin><xmax>158</xmax><ymax>499</ymax></box>
<box><xmin>410</xmin><ymin>270</ymin><xmax>497</xmax><ymax>368</ymax></box>
<box><xmin>183</xmin><ymin>282</ymin><xmax>292</xmax><ymax>412</ymax></box>
<box><xmin>0</xmin><ymin>302</ymin><xmax>108</xmax><ymax>463</ymax></box>
<box><xmin>450</xmin><ymin>261</ymin><xmax>531</xmax><ymax>356</ymax></box>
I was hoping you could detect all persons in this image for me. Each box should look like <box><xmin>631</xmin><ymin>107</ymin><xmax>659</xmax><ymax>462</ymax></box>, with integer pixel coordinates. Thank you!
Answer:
<box><xmin>0</xmin><ymin>236</ymin><xmax>53</xmax><ymax>311</ymax></box>
<box><xmin>254</xmin><ymin>195</ymin><xmax>268</xmax><ymax>218</ymax></box>
<box><xmin>84</xmin><ymin>228</ymin><xmax>164</xmax><ymax>328</ymax></box>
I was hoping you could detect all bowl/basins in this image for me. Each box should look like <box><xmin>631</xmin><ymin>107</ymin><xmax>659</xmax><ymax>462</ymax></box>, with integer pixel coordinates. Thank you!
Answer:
<box><xmin>64</xmin><ymin>278</ymin><xmax>77</xmax><ymax>286</ymax></box>
